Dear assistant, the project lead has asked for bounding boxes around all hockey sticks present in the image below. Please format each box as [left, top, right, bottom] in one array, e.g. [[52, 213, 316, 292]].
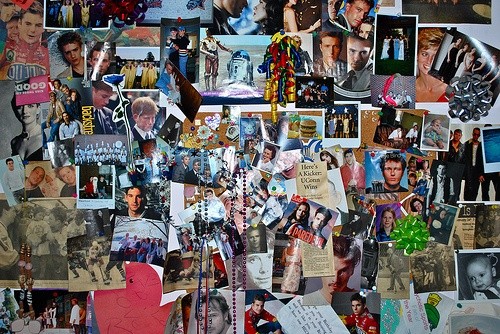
[[386, 264, 398, 295], [83, 259, 95, 282], [99, 266, 113, 283]]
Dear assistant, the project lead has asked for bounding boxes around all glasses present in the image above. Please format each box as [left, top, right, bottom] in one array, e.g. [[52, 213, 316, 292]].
[[472, 133, 481, 137]]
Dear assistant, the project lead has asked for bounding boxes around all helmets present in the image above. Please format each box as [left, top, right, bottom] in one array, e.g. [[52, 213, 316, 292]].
[[92, 241, 97, 248]]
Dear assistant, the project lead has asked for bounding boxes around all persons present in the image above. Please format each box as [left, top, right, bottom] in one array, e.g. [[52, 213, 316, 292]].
[[0, 0, 500, 334]]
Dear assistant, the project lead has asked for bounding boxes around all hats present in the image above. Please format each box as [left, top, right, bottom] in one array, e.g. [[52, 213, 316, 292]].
[[178, 26, 186, 30]]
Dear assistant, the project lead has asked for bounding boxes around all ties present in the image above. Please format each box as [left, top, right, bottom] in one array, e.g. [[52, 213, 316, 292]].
[[145, 134, 149, 140], [99, 111, 103, 127], [341, 70, 356, 91]]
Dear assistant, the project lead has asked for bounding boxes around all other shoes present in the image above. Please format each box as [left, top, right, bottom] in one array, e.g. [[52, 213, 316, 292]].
[[92, 278, 97, 282], [73, 274, 80, 279], [121, 279, 126, 283]]
